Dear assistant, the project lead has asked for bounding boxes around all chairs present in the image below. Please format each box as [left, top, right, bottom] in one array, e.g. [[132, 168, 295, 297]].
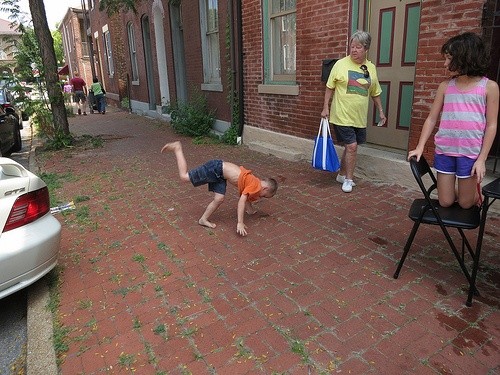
[[393, 154, 480, 296]]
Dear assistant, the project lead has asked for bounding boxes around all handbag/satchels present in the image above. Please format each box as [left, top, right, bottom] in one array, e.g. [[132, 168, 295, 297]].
[[311, 116, 339, 173], [100, 83, 106, 94]]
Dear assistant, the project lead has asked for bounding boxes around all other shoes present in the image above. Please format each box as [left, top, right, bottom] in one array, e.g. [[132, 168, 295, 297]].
[[83, 111, 88, 115], [78, 109, 81, 115], [473, 174, 482, 209]]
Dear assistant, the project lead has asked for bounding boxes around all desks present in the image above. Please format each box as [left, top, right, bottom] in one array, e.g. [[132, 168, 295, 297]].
[[466, 178, 500, 307]]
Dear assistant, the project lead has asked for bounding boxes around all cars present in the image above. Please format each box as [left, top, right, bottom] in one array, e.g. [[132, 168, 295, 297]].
[[0, 157, 62, 300], [0, 76, 33, 157]]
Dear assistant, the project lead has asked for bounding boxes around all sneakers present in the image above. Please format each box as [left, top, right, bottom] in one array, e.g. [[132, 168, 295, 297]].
[[336, 175, 354, 193]]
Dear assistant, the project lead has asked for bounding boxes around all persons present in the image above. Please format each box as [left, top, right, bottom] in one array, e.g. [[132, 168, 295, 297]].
[[89, 78, 106, 115], [407, 32, 500, 209], [321, 30, 386, 193], [64, 81, 72, 103], [162, 140, 278, 237], [70, 72, 87, 116]]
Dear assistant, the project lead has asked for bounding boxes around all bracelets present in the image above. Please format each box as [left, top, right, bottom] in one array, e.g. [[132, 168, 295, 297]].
[[378, 109, 383, 112]]
